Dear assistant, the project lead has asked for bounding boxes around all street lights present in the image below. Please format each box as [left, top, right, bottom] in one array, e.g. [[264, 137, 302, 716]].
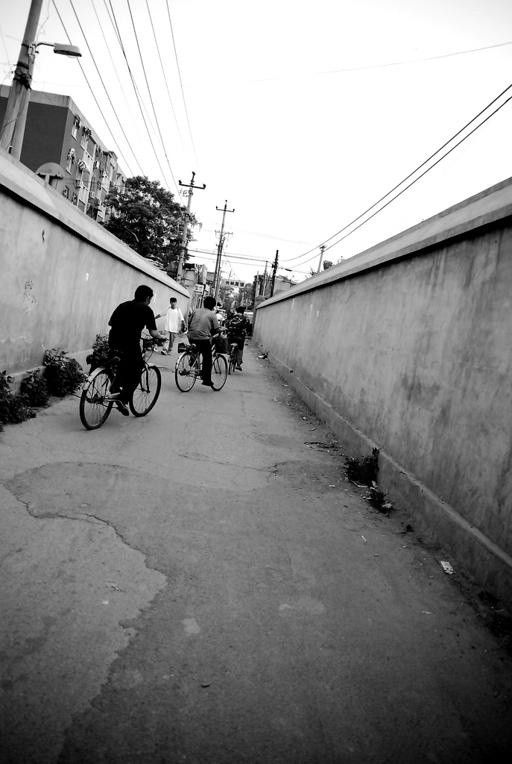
[[2, 42, 82, 156]]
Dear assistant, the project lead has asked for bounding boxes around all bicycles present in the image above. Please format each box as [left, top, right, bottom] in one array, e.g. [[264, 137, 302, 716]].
[[174, 328, 228, 393], [79, 337, 167, 431], [228, 342, 238, 375]]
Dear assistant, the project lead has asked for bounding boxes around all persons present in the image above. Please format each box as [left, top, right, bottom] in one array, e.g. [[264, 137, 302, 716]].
[[241, 306, 256, 345], [106, 284, 167, 417], [215, 310, 225, 327], [222, 305, 253, 371], [185, 296, 229, 386], [154, 297, 186, 355]]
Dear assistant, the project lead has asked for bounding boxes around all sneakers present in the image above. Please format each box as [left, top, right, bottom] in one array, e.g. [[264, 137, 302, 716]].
[[116, 399, 129, 416], [203, 379, 213, 386], [161, 349, 171, 355], [236, 366, 242, 371]]
